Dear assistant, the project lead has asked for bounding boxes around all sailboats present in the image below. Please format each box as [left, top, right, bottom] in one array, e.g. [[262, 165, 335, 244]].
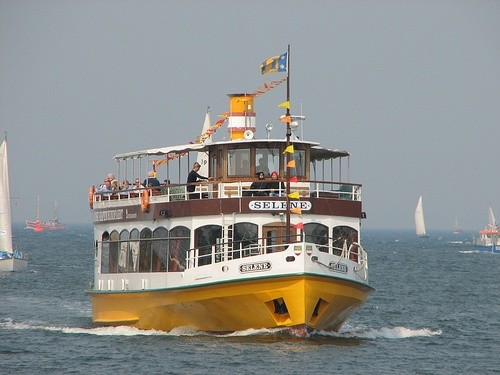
[[413, 193, 431, 239], [0, 129, 30, 273]]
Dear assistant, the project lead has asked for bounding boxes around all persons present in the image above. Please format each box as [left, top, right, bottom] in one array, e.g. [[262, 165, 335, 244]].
[[255, 158, 270, 176], [266, 171, 285, 197], [95, 171, 170, 197], [187, 162, 213, 199], [250, 171, 270, 196]]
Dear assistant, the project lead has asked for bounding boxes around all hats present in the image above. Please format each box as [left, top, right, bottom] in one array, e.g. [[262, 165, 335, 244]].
[[272, 171, 278, 176]]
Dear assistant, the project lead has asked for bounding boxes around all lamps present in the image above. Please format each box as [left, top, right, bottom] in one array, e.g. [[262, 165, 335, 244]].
[[267, 122, 273, 140]]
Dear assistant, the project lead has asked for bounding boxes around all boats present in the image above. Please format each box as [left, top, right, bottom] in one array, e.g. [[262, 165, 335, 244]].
[[25, 195, 64, 233], [81, 42, 377, 335], [471, 222, 500, 253]]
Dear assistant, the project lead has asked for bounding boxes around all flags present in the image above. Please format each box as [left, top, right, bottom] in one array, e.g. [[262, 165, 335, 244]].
[[260, 52, 288, 75]]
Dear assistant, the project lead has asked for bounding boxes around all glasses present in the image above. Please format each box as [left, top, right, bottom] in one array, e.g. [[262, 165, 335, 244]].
[[136, 182, 139, 183]]
